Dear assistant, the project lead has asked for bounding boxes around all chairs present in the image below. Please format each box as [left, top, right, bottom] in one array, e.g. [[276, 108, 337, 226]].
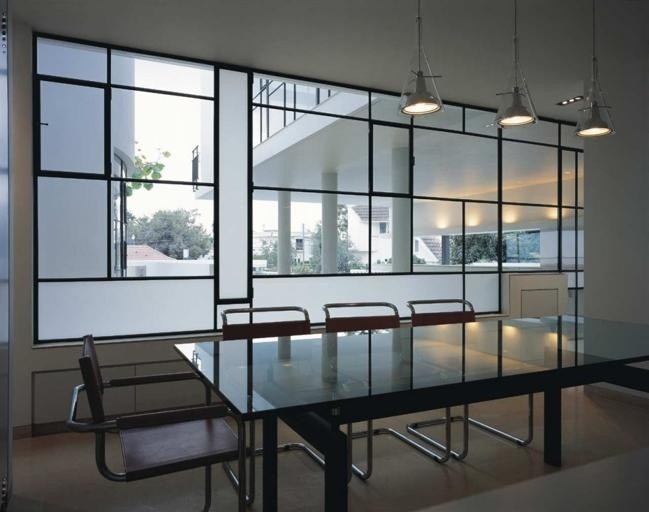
[[69, 331, 244, 512], [221, 306, 352, 505], [405, 299, 534, 461], [322, 302, 451, 481]]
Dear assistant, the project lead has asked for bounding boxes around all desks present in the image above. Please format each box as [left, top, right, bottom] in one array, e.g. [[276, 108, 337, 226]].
[[172, 315, 647, 512]]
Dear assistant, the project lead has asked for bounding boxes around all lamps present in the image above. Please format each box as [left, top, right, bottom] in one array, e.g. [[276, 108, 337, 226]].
[[572, 1, 614, 138], [396, 0, 443, 117], [499, 1, 539, 128]]
